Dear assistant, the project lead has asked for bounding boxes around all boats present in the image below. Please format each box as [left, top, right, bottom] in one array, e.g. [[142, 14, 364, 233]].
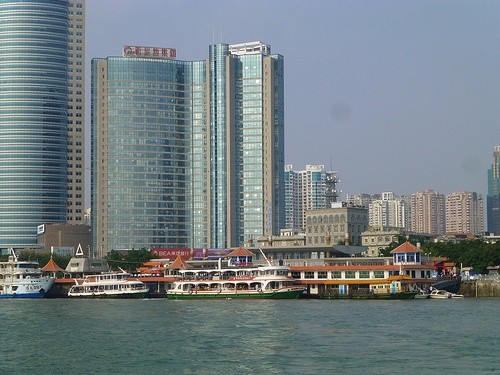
[[0, 249, 57, 298], [428, 290, 465, 300], [64, 273, 152, 299], [281, 263, 422, 301], [165, 268, 308, 299]]
